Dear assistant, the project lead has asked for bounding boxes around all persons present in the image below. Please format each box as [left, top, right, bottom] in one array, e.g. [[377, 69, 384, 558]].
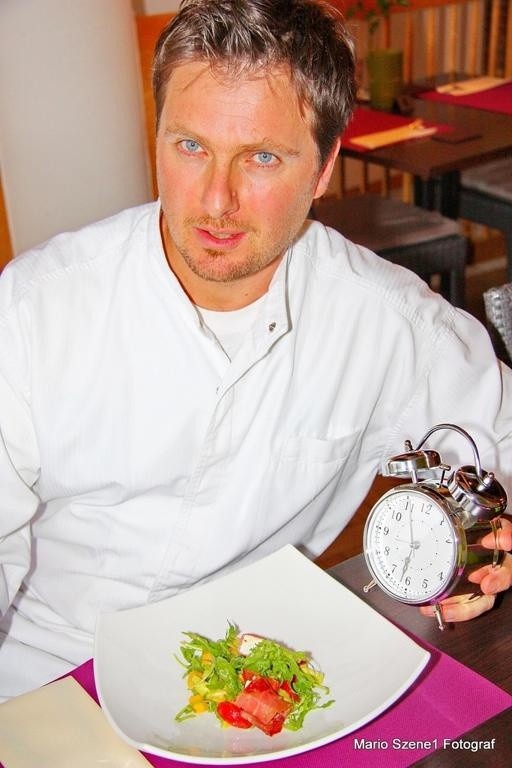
[[0, 0, 512, 705]]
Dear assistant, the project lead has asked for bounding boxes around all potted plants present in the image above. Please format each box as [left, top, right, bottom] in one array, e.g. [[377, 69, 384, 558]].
[[348, 0, 410, 112]]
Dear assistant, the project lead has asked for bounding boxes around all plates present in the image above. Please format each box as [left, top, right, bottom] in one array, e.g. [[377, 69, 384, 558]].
[[94, 542, 433, 765]]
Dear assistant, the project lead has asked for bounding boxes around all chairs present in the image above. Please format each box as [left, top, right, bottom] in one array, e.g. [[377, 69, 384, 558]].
[[312, 193, 474, 312], [424, 158, 512, 288]]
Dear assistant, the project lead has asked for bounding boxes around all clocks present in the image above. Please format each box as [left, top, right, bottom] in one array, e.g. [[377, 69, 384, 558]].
[[363, 423, 507, 631]]
